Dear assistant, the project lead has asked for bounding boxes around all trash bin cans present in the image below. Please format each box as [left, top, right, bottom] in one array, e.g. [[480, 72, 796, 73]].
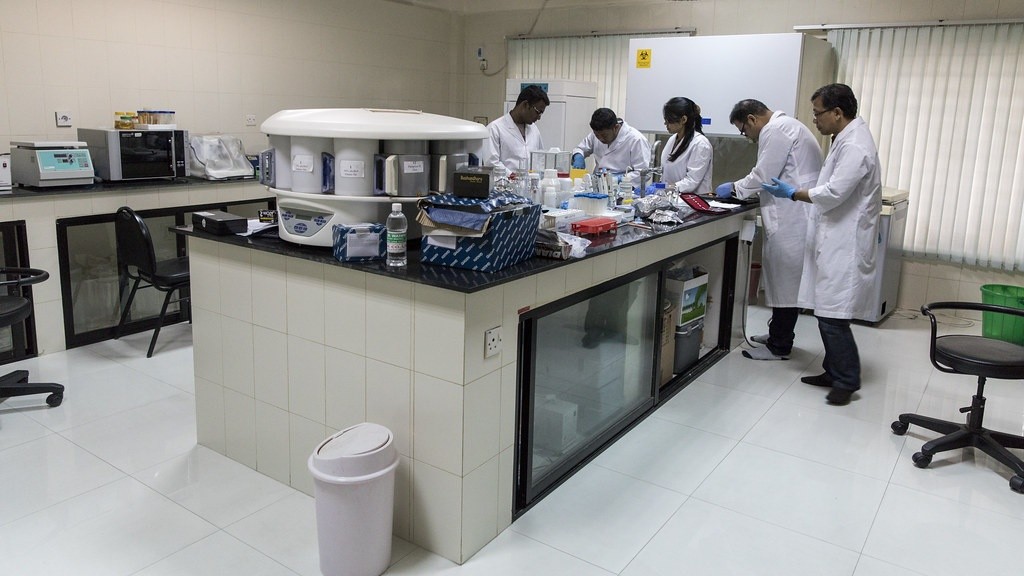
[[979, 283, 1024, 347], [307, 421, 400, 576]]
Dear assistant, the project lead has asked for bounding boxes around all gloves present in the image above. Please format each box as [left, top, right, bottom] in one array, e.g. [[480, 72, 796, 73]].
[[634, 186, 641, 195], [714, 182, 734, 198], [762, 177, 796, 201], [572, 154, 586, 169], [617, 175, 622, 185], [646, 181, 656, 195]]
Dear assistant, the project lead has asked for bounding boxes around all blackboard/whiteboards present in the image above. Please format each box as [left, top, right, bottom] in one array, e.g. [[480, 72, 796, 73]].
[[625, 33, 805, 139]]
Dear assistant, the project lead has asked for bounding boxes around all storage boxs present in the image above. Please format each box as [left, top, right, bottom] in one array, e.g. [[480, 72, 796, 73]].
[[665, 265, 709, 327], [192, 210, 247, 234], [415, 189, 542, 273], [658, 308, 676, 388], [332, 223, 387, 262]]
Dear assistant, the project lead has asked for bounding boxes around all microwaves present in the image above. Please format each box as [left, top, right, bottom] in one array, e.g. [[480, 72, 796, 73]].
[[77, 126, 187, 182]]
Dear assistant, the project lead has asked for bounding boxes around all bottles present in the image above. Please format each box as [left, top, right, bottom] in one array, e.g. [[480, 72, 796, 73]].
[[479, 167, 583, 209], [114, 112, 139, 130], [385, 203, 408, 267], [620, 178, 632, 201], [654, 183, 666, 200]]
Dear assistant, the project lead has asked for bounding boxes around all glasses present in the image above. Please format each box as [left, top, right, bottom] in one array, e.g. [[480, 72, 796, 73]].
[[740, 113, 755, 137], [663, 116, 682, 124], [812, 106, 844, 120], [532, 104, 543, 117]]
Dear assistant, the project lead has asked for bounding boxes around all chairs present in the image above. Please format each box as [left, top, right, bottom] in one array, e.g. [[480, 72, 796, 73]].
[[889, 302, 1024, 493], [0, 265, 65, 407], [114, 206, 191, 357]]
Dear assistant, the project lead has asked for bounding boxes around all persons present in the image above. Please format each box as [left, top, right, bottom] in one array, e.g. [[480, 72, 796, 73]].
[[715, 98, 826, 361], [760, 84, 882, 406], [483, 85, 550, 180], [581, 274, 640, 350], [659, 97, 714, 194], [571, 108, 652, 188]]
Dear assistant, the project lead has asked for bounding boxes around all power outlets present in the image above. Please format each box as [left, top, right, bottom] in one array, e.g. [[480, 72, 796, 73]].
[[55, 111, 72, 126], [474, 117, 488, 126], [484, 326, 503, 359], [245, 115, 256, 125], [480, 61, 487, 69]]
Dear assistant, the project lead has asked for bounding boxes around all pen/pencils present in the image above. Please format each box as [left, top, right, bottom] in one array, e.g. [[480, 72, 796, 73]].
[[627, 223, 652, 230]]
